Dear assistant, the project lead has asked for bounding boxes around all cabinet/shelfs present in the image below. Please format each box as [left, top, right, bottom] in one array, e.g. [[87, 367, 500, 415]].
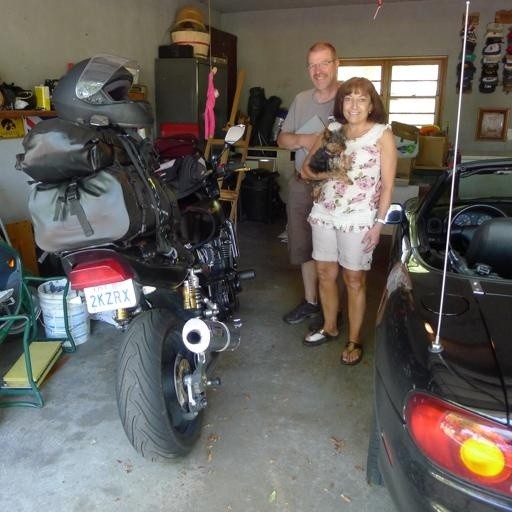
[[155, 59, 228, 143]]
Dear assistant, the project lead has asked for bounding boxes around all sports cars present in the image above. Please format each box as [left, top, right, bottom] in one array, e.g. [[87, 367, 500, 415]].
[[366, 159, 511, 512]]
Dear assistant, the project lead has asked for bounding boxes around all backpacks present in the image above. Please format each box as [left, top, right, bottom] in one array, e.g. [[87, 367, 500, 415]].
[[16, 119, 150, 178]]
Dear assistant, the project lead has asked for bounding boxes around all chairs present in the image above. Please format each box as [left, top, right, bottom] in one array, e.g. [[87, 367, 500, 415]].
[[0, 238, 77, 410], [468, 216, 511, 279]]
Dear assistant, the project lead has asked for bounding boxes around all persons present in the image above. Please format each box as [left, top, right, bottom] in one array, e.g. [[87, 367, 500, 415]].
[[276, 43, 346, 331], [302, 76, 396, 366]]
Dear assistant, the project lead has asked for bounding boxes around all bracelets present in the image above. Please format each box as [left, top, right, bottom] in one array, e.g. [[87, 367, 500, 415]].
[[375, 217, 386, 225]]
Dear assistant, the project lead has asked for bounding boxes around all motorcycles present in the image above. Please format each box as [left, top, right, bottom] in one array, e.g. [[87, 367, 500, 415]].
[[56, 123, 257, 464]]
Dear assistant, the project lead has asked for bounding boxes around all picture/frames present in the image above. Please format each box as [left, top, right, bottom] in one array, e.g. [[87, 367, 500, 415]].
[[477, 107, 510, 141]]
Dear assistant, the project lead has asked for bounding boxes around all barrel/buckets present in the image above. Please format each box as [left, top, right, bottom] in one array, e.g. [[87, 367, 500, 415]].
[[38, 280, 91, 349]]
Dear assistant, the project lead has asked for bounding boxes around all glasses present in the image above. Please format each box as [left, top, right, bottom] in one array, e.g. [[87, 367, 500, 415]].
[[307, 59, 335, 71]]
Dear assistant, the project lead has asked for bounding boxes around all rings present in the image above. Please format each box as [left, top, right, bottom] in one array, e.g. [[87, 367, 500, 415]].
[[373, 245, 376, 248]]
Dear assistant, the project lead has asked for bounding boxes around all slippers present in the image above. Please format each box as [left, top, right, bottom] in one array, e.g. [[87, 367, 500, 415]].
[[340, 341, 364, 365], [303, 328, 340, 347]]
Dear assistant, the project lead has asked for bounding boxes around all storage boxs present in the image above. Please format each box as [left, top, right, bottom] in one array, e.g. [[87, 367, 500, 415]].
[[391, 122, 450, 179]]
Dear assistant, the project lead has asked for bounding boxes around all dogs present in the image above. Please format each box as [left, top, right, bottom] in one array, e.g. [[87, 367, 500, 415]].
[[301, 127, 354, 202]]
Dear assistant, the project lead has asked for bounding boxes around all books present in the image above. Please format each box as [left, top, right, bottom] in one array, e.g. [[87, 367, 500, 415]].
[[294, 114, 325, 156]]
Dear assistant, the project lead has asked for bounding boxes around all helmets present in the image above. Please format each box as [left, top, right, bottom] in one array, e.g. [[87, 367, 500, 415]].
[[50, 52, 154, 132]]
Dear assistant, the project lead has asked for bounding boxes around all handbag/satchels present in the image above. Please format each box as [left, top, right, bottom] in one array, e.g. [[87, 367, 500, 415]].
[[28, 165, 182, 257]]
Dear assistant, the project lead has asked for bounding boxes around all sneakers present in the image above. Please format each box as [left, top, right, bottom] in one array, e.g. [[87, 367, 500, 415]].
[[309, 307, 345, 331], [283, 295, 322, 325]]
